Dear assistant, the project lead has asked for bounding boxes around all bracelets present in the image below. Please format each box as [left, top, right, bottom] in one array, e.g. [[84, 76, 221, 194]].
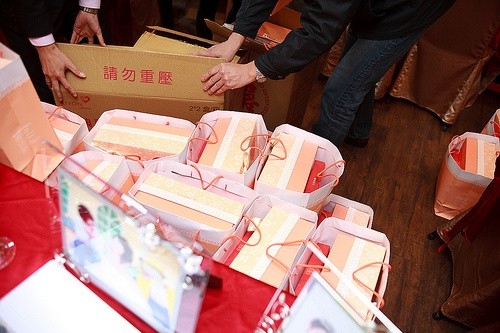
[[80, 6, 98, 14]]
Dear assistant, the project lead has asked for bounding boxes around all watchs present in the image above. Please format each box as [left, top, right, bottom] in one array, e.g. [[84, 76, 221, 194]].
[[256, 68, 266, 83]]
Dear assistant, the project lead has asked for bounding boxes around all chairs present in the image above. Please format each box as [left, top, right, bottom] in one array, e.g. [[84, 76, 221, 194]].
[[320, 22, 397, 104], [384, 0, 500, 134], [426, 174, 500, 333]]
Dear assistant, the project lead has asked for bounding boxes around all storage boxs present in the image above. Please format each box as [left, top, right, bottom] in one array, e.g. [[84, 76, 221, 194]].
[[46, 112, 81, 143], [228, 207, 315, 291], [77, 160, 118, 193], [50, 41, 229, 128], [204, 17, 315, 131], [304, 159, 326, 194], [133, 26, 250, 112], [133, 171, 245, 231], [331, 203, 370, 229], [459, 137, 496, 179], [256, 133, 319, 192], [321, 232, 388, 322], [265, 0, 305, 31], [224, 230, 256, 266], [93, 117, 193, 153], [196, 117, 259, 174], [317, 209, 333, 228], [494, 110, 500, 133], [295, 242, 331, 296]]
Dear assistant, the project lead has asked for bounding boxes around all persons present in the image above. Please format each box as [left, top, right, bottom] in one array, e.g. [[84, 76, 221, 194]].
[[187, 0, 456, 151], [73, 205, 100, 269], [111, 234, 132, 263], [0, 0, 106, 101]]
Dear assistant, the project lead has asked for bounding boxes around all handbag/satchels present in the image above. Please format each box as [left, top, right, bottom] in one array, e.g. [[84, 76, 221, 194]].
[[0, 44, 395, 333], [433, 108, 500, 220]]
